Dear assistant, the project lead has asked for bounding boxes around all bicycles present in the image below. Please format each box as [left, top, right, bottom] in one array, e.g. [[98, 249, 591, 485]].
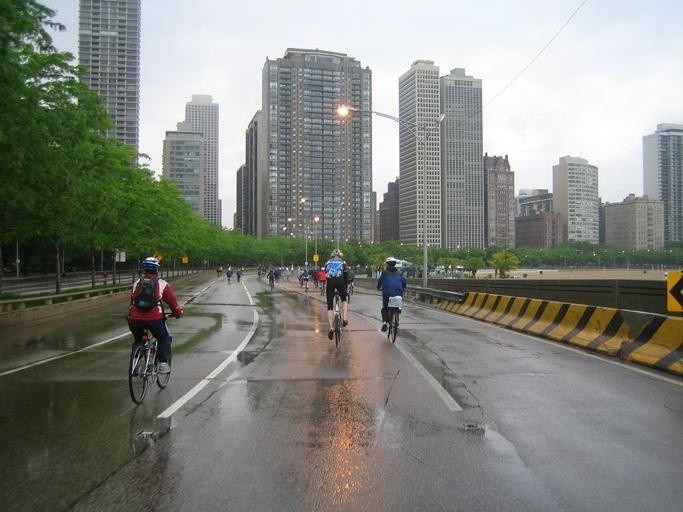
[[268, 279, 276, 292], [129, 313, 174, 404], [379, 289, 402, 342], [228, 277, 232, 284], [328, 288, 347, 349], [300, 278, 354, 303]]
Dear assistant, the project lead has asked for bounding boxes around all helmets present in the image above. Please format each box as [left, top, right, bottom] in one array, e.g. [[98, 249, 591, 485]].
[[330, 249, 344, 260], [384, 256, 398, 264], [141, 256, 161, 273], [320, 267, 325, 271]]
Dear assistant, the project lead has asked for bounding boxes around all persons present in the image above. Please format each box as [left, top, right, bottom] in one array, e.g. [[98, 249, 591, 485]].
[[213, 262, 478, 295], [124, 257, 184, 375], [322, 248, 350, 340], [376, 257, 407, 332]]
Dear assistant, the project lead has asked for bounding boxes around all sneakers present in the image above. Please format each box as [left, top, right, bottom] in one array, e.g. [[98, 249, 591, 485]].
[[328, 320, 348, 340], [159, 362, 171, 374], [381, 324, 388, 331]]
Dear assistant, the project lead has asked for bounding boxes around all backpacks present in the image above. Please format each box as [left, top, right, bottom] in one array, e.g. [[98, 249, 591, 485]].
[[132, 277, 162, 311], [324, 261, 343, 279]]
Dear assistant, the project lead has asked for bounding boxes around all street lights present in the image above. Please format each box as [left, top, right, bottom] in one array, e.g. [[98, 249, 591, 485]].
[[338, 106, 428, 288], [288, 218, 308, 269], [302, 198, 340, 250]]
